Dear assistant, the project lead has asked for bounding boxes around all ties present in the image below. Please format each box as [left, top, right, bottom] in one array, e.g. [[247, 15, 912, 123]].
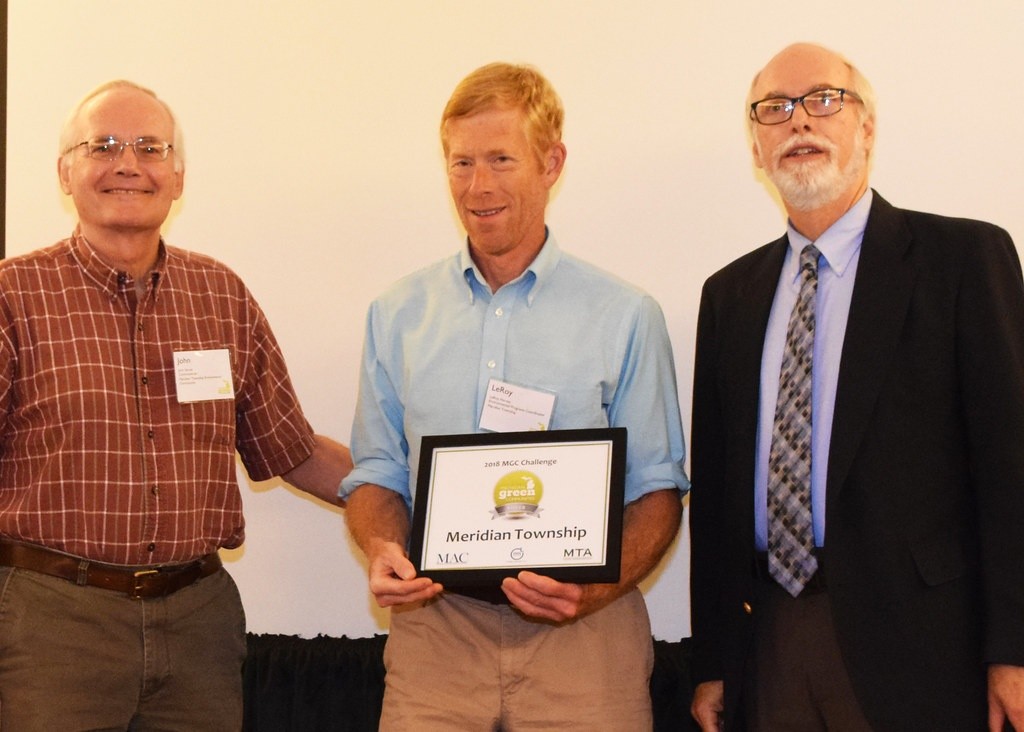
[[766, 245, 823, 597]]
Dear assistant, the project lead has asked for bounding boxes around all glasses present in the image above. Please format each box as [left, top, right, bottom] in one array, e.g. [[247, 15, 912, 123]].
[[749, 85, 857, 125], [61, 135, 180, 164]]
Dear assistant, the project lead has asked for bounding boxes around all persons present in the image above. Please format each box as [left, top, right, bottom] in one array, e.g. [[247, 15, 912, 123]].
[[0, 81, 353, 732], [690, 42, 1024, 732], [337, 63, 691, 732]]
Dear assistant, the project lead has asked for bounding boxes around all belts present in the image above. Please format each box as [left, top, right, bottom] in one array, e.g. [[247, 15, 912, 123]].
[[0, 540, 220, 600]]
